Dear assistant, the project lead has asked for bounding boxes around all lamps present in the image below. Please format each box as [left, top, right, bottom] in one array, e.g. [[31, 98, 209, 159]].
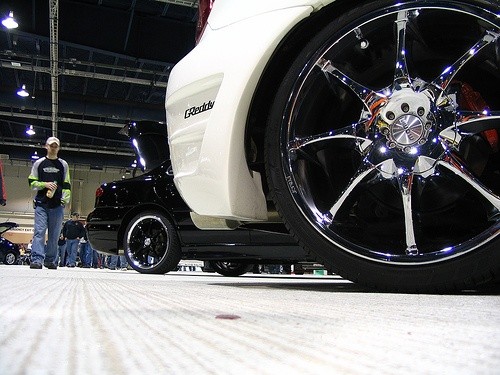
[[31, 151, 40, 159], [17, 83, 29, 96], [26, 125, 35, 135], [1, 10, 18, 29]]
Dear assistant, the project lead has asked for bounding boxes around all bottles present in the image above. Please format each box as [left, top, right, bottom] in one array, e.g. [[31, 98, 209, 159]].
[[46, 181, 58, 198]]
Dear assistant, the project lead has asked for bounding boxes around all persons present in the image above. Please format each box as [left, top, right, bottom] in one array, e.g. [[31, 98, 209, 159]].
[[63, 212, 87, 268], [16, 220, 336, 275], [27, 136, 72, 269]]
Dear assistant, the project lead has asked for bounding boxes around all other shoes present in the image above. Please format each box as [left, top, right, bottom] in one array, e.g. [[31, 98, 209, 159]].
[[44, 260, 57, 269], [30, 261, 42, 269]]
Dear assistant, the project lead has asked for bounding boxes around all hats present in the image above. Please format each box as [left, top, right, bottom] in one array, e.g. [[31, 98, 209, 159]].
[[46, 136, 60, 147]]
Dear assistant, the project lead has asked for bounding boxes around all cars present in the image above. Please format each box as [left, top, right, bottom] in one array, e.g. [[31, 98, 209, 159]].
[[0, 221, 21, 266], [80, 121, 320, 275], [164, 0, 500, 295]]
